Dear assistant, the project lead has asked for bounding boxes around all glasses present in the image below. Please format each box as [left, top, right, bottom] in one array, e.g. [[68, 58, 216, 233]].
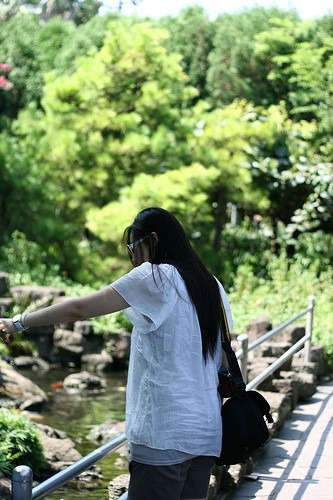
[[127, 236, 149, 257]]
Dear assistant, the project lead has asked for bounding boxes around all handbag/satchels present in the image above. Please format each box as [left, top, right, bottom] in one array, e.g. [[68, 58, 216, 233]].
[[216, 391, 273, 467]]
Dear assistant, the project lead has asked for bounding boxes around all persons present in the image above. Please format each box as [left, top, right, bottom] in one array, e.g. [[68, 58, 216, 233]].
[[0, 208, 233, 500]]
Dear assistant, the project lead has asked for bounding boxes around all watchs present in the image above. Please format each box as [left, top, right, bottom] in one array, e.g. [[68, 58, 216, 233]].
[[12, 314, 25, 331]]
